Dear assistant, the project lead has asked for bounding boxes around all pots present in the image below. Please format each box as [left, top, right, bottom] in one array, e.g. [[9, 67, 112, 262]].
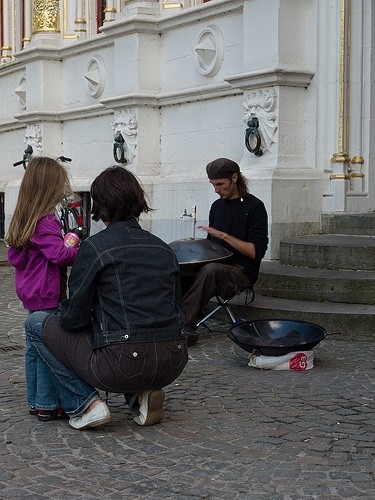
[[228, 319, 342, 357]]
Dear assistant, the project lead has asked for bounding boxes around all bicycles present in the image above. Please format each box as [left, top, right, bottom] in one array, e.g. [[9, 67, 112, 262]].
[[13, 155, 90, 242]]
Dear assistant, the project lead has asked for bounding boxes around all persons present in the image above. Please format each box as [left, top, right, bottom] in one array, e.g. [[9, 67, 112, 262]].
[[179, 158, 269, 347], [5, 156, 81, 421], [24, 165, 188, 430]]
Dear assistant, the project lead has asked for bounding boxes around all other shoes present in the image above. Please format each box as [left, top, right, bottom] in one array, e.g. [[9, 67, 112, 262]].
[[180, 326, 199, 347], [38, 408, 69, 421], [132, 389, 165, 426], [30, 407, 38, 414], [69, 401, 112, 430]]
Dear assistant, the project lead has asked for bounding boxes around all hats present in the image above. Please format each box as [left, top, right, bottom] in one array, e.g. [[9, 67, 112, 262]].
[[206, 158, 240, 178]]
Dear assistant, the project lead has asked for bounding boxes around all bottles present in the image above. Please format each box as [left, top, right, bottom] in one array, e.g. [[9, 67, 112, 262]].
[[64, 227, 84, 246]]
